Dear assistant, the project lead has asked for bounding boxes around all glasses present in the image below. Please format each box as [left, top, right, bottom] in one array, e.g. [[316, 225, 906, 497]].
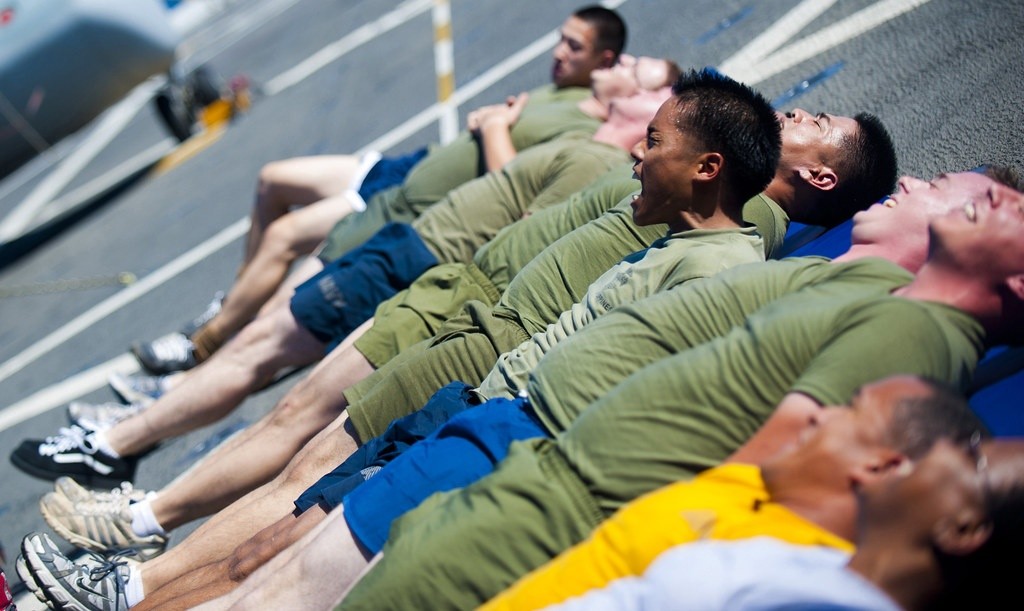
[[970, 430, 1001, 515]]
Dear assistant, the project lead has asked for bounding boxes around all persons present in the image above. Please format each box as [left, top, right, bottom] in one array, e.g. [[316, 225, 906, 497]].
[[476, 373, 973, 609], [36, 106, 899, 565], [16, 67, 787, 611], [333, 183, 1024, 610], [64, 51, 684, 436], [133, 4, 630, 375], [10, 84, 677, 488], [184, 162, 1018, 611], [548, 435, 1024, 607]]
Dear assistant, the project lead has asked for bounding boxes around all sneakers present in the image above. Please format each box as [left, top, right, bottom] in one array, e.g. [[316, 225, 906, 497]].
[[68, 401, 137, 430], [16, 531, 128, 611], [56, 476, 152, 503], [129, 331, 199, 372], [40, 492, 170, 563], [182, 289, 224, 338], [11, 430, 134, 487], [109, 368, 183, 407]]
